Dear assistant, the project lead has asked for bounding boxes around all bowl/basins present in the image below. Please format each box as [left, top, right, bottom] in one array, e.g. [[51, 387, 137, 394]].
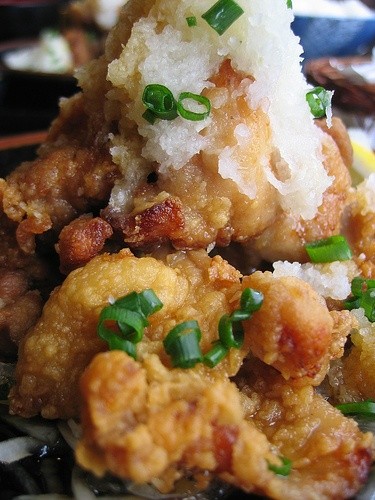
[[0, 39, 81, 104], [0, 130, 375, 500]]
